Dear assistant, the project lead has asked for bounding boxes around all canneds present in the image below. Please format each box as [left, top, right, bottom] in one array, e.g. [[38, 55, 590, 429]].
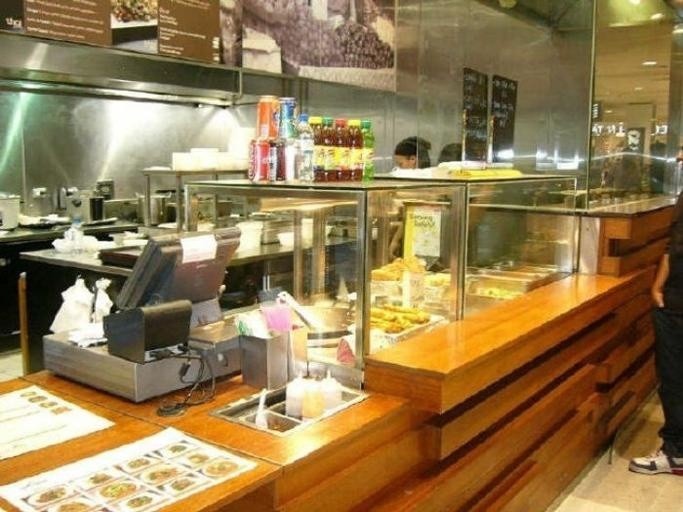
[[249, 95, 296, 182]]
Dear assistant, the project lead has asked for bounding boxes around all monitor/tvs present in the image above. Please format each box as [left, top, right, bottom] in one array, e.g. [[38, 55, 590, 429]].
[[116, 228, 241, 311]]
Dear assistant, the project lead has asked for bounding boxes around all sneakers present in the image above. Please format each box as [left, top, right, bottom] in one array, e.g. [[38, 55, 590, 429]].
[[628, 449, 681, 477]]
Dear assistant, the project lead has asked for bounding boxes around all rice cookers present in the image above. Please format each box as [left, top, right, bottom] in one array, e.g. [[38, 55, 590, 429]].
[[0, 191, 25, 231]]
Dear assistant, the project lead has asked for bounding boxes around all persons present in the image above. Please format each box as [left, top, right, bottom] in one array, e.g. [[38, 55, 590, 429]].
[[629, 145, 683, 475], [388, 136, 430, 262]]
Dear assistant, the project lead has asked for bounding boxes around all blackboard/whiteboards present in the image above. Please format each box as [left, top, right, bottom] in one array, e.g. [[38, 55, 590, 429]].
[[462, 67, 488, 163], [492, 75, 518, 163]]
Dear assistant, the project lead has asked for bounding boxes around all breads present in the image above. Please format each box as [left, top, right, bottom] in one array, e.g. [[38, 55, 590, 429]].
[[362, 258, 472, 334]]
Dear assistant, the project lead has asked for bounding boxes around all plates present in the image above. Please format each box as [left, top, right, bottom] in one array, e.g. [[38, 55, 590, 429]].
[[20, 390, 68, 413], [109, 3, 163, 30], [30, 441, 238, 512]]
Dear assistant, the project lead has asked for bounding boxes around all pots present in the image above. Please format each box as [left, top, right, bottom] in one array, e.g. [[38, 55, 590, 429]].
[[134, 194, 172, 226]]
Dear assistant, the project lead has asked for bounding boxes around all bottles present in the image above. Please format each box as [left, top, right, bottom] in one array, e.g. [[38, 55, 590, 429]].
[[72, 214, 80, 228], [91, 197, 103, 221], [52, 187, 57, 210], [59, 186, 66, 210], [248, 94, 378, 181]]
